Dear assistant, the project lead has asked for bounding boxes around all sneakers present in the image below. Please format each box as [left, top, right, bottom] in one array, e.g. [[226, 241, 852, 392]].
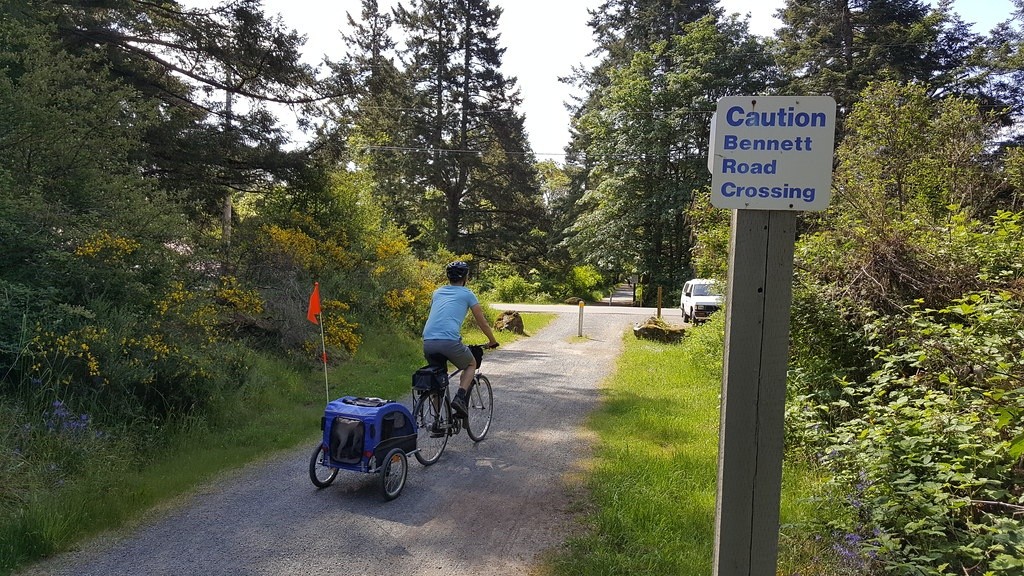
[[432, 417, 446, 434], [451, 396, 469, 417]]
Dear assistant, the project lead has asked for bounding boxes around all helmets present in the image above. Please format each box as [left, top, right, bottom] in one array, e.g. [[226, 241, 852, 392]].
[[447, 261, 468, 278]]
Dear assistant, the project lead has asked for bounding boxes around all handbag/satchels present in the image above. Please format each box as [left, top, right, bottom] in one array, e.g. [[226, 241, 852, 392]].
[[411, 364, 449, 389]]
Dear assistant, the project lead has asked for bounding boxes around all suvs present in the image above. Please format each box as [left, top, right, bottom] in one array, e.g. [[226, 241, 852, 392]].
[[679, 278, 726, 327]]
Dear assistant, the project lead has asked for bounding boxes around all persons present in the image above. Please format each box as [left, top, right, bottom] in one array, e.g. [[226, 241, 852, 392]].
[[422, 262, 497, 437]]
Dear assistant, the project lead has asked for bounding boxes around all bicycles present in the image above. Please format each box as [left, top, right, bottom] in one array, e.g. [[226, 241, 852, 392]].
[[411, 343, 500, 466]]
